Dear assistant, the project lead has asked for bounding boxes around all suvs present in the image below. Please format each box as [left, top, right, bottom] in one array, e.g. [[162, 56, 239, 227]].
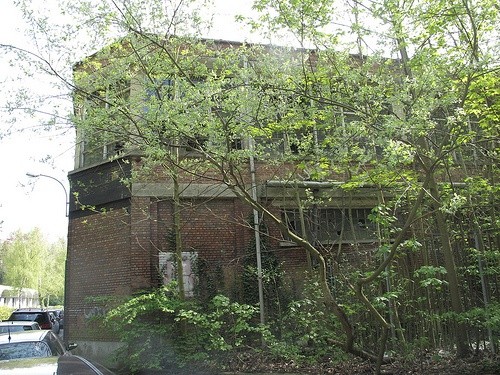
[[8, 309, 61, 332]]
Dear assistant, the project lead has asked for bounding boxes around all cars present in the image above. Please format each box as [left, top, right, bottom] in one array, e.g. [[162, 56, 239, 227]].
[[1, 321, 42, 335], [45, 309, 61, 327], [0, 356, 118, 375], [55, 310, 64, 328], [0, 329, 77, 362]]
